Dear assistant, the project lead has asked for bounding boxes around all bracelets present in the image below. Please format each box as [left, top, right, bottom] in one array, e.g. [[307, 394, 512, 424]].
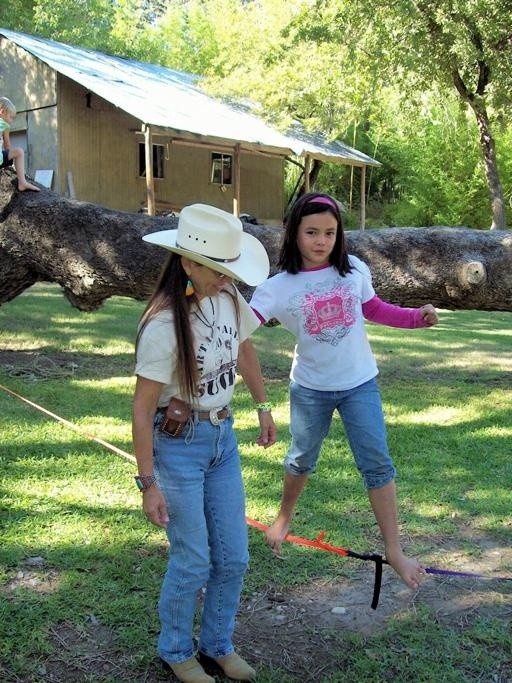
[[135, 474, 156, 492], [255, 401, 274, 412]]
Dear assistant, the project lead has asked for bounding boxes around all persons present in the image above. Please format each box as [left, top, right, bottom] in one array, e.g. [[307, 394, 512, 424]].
[[247, 193, 437, 587], [0, 96, 40, 191], [132, 201, 276, 683]]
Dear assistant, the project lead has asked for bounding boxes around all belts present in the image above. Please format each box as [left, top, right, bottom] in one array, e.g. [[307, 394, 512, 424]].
[[156, 407, 230, 421]]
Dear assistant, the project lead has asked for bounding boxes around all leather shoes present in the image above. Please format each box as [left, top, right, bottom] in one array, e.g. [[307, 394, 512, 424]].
[[214, 651, 256, 680], [169, 655, 216, 683]]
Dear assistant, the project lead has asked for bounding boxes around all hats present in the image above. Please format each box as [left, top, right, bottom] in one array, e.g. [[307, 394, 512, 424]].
[[140, 202, 270, 287]]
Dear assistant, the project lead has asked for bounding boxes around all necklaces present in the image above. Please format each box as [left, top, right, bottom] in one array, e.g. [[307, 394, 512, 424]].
[[194, 295, 215, 340]]
